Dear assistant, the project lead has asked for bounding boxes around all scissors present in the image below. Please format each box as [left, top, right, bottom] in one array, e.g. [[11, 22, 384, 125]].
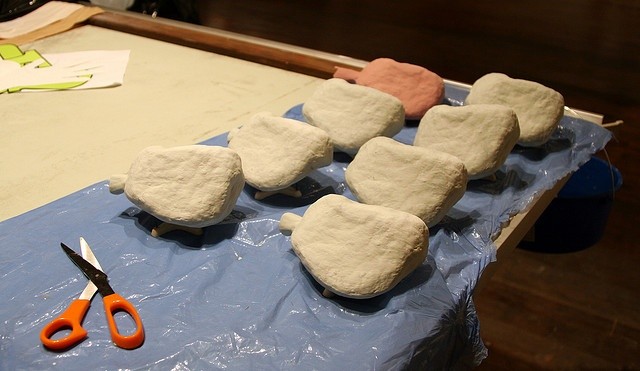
[[39, 238, 144, 351]]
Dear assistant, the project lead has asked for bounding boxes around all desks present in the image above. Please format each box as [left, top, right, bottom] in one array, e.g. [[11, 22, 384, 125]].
[[0, 1, 604, 371]]
